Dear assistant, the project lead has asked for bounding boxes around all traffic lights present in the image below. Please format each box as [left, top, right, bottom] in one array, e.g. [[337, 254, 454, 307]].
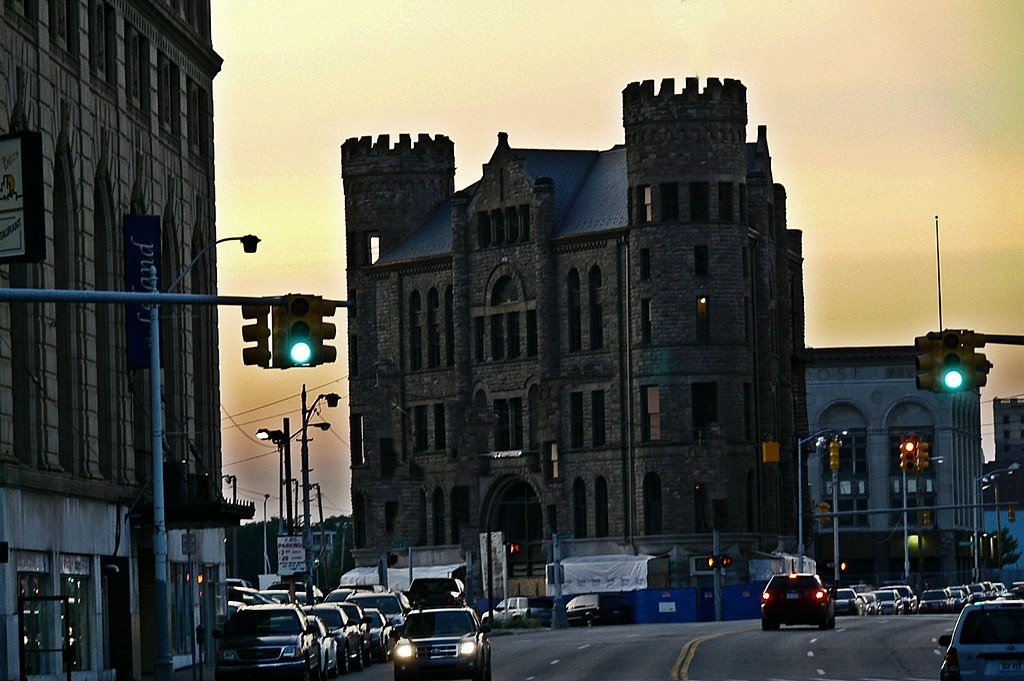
[[900, 439, 929, 473], [914, 330, 989, 392], [242, 290, 336, 370], [819, 503, 830, 522], [708, 555, 732, 568], [504, 540, 521, 555]]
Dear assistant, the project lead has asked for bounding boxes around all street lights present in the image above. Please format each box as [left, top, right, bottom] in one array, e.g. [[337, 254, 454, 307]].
[[148, 235, 262, 680], [301, 382, 343, 605], [902, 454, 945, 584], [278, 422, 332, 535], [979, 462, 1021, 570], [262, 493, 271, 574], [797, 425, 848, 573]]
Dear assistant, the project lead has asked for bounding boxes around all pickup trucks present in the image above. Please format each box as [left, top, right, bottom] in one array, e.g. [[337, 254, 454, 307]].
[[480, 596, 551, 626]]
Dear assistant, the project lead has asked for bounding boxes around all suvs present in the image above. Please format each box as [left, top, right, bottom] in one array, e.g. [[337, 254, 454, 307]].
[[761, 574, 835, 631], [849, 581, 1024, 617], [836, 588, 860, 616], [565, 592, 631, 628], [393, 605, 494, 681], [408, 577, 465, 611], [939, 599, 1024, 681], [225, 577, 411, 681]]
